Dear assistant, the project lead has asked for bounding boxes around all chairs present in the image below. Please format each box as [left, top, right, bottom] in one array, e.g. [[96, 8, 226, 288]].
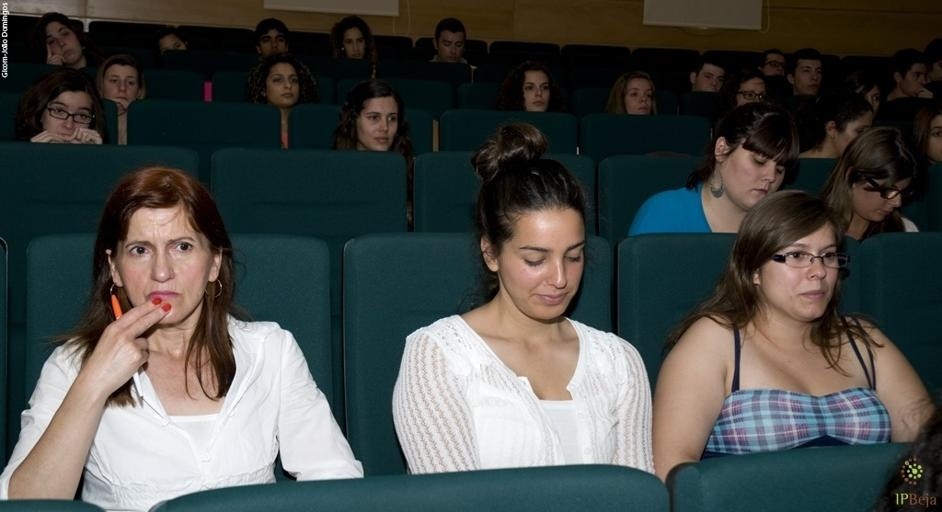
[[2, 16, 942, 235], [619, 234, 858, 464], [28, 245, 338, 476], [856, 232, 941, 408], [344, 241, 612, 475], [146, 464, 669, 512], [667, 444, 906, 512]]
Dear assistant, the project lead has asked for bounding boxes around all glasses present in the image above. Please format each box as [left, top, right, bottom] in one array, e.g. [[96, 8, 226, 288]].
[[48, 106, 95, 126], [737, 89, 767, 101], [763, 60, 785, 68], [869, 178, 916, 199], [773, 250, 849, 269]]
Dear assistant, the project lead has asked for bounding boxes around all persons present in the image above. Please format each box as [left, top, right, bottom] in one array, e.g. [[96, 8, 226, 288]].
[[15, 1, 942, 236], [393, 119, 659, 475], [654, 189, 938, 485], [865, 381, 942, 512], [0, 164, 365, 511]]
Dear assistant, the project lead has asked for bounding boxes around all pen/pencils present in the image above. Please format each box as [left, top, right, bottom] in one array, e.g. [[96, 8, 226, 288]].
[[111, 294, 144, 401]]
